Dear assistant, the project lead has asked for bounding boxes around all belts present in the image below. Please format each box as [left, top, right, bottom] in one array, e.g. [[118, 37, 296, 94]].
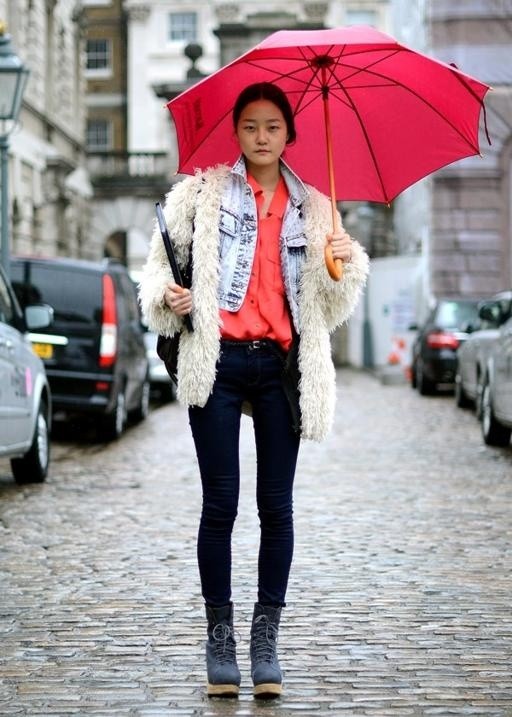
[[220, 338, 277, 351]]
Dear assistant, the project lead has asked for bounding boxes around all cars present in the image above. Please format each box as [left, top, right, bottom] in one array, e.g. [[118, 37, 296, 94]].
[[0, 264, 52, 484], [7, 258, 154, 438], [406, 287, 512, 442]]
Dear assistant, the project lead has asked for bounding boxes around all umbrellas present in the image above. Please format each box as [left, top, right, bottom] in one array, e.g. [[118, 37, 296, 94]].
[[162, 26, 493, 280]]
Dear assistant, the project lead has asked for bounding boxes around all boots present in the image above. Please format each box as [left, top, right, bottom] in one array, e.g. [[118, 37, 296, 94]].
[[250, 602, 282, 697], [205, 600, 241, 697]]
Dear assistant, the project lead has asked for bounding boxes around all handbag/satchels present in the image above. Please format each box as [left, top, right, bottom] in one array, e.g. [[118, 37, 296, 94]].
[[157, 254, 192, 386]]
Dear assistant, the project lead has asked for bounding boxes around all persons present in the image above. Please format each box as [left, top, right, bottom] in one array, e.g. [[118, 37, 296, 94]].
[[137, 82, 370, 697]]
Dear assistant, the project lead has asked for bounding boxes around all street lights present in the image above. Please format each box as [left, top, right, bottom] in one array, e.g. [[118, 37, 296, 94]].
[[0, 28, 29, 284]]
